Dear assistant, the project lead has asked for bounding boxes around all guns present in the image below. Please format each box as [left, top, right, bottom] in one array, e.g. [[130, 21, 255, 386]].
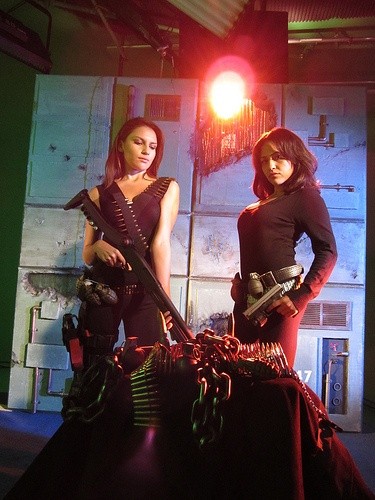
[[242, 281, 284, 328], [64, 189, 195, 341]]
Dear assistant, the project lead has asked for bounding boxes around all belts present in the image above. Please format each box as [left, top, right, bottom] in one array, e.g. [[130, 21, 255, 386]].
[[259, 265, 304, 290]]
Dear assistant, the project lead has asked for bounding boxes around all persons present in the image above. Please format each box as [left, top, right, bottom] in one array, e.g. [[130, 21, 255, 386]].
[[78, 117, 179, 365], [234, 128, 338, 371]]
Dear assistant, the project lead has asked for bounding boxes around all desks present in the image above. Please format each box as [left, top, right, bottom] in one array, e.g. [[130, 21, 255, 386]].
[[0, 348, 375, 500]]
